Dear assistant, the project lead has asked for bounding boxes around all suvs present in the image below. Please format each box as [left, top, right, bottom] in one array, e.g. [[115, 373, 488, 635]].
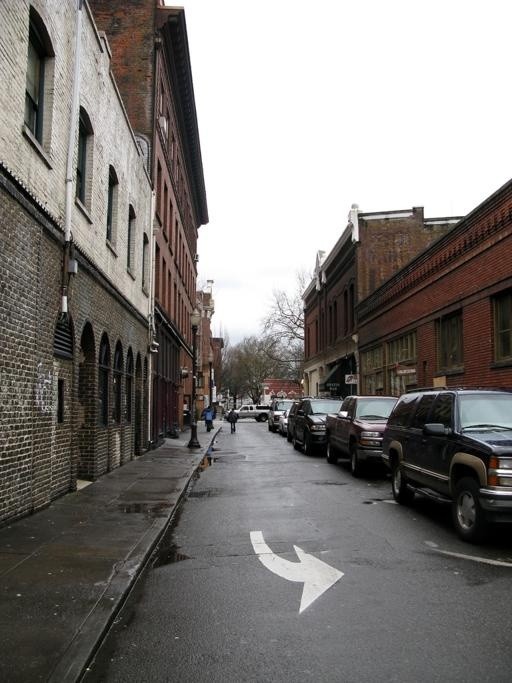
[[292, 398, 345, 456], [287, 401, 299, 443], [268, 398, 292, 433], [224, 404, 271, 422], [380, 384, 512, 544]]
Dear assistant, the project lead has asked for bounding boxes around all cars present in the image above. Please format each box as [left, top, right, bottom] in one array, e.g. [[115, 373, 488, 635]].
[[279, 410, 289, 437]]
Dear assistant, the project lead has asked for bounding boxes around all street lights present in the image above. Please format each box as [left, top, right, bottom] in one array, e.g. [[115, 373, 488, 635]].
[[189, 308, 201, 448], [208, 348, 213, 405]]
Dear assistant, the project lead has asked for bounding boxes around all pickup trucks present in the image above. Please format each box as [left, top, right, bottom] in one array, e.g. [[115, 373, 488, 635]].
[[325, 396, 399, 477]]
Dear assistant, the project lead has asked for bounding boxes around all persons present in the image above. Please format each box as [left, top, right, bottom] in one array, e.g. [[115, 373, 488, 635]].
[[226, 407, 239, 433], [203, 405, 214, 432]]
